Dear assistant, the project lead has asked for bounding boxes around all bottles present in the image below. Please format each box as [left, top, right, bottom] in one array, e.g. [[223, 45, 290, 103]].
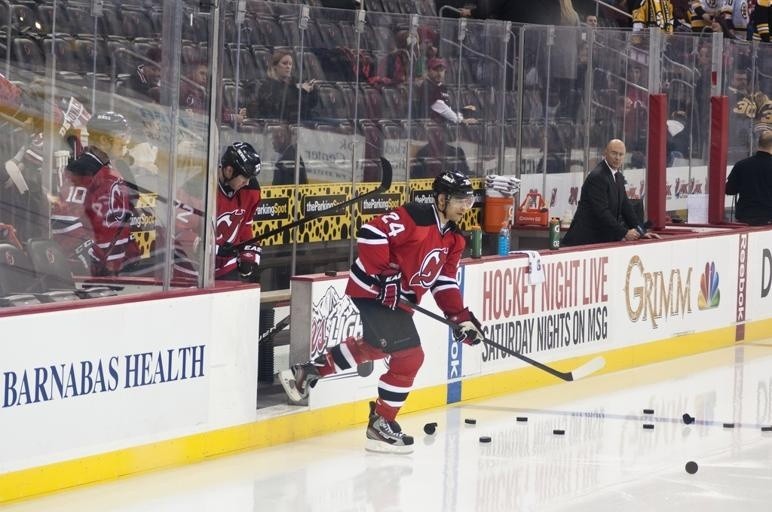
[[498, 222, 512, 257], [470, 221, 483, 257], [549, 216, 561, 250]]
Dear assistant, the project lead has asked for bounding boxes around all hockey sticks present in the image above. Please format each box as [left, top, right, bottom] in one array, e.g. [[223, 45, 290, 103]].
[[398, 296, 605, 381], [219, 157, 393, 255], [5, 160, 30, 250], [97, 161, 138, 276]]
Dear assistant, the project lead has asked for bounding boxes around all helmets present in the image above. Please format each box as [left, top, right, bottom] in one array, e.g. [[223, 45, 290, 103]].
[[432, 170, 474, 200], [225, 141, 261, 176], [87, 111, 131, 134]]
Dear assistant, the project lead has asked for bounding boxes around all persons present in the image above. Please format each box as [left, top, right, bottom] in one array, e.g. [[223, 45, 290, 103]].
[[0, 0, 772, 288], [290, 169, 485, 447]]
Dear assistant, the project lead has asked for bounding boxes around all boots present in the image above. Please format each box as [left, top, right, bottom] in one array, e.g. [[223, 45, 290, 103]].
[[367, 414, 414, 446], [292, 362, 321, 400]]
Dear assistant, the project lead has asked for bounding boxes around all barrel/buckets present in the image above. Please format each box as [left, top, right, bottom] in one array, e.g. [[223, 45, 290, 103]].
[[484, 195, 515, 234]]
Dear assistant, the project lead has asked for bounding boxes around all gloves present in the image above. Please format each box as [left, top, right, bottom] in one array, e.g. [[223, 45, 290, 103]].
[[237, 244, 262, 282], [76, 240, 107, 274], [447, 307, 484, 345], [376, 269, 410, 311], [196, 240, 234, 262]]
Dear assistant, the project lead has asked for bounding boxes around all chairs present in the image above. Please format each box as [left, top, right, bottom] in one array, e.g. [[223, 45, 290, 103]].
[[0, 243, 40, 296], [0, 1, 771, 183], [26, 237, 75, 290]]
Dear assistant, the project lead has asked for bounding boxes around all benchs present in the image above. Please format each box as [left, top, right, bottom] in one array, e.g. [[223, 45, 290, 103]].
[[258, 240, 360, 268], [498, 227, 572, 250]]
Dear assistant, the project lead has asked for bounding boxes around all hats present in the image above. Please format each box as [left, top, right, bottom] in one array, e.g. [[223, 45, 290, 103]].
[[428, 57, 448, 69]]
